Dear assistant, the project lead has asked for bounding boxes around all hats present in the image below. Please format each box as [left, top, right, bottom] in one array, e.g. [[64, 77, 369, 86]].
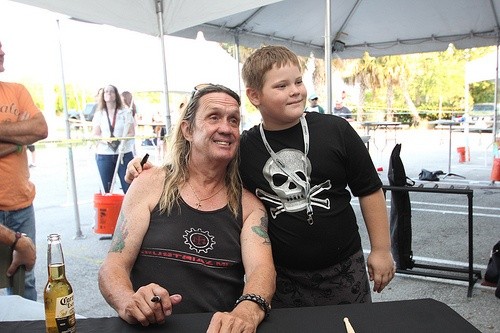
[[309, 93, 319, 101]]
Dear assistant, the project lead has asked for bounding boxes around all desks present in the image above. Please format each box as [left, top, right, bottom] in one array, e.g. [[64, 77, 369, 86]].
[[360, 122, 402, 152], [1, 297, 483, 333]]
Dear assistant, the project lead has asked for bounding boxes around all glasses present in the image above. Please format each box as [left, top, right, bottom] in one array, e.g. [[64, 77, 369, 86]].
[[192, 83, 241, 105]]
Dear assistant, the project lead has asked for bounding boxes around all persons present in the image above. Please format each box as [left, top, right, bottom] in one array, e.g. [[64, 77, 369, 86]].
[[332, 101, 353, 122], [304, 94, 324, 114], [124, 45, 395, 308], [90, 85, 138, 194], [0, 41, 47, 301], [98, 83, 278, 333]]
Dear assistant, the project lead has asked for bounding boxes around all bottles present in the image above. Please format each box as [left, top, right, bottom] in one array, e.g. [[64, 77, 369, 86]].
[[8, 233, 27, 299], [43, 232, 80, 333]]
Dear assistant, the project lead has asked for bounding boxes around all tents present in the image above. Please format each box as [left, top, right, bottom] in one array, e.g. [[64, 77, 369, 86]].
[[463, 50, 500, 163], [9, 0, 500, 240]]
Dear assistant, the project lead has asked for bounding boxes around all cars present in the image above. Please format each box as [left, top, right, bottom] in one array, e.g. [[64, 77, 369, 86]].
[[68, 104, 97, 130], [468, 103, 496, 130]]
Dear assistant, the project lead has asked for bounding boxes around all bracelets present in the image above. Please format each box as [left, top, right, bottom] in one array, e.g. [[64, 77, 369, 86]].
[[234, 294, 272, 319], [17, 144, 23, 153], [12, 232, 21, 247]]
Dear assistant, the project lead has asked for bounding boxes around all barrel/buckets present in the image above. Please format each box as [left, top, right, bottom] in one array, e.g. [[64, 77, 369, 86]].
[[456, 146, 468, 162], [91, 192, 125, 235]]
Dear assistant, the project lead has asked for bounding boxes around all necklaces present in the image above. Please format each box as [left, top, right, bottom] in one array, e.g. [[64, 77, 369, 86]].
[[186, 178, 227, 210], [260, 114, 314, 225]]
